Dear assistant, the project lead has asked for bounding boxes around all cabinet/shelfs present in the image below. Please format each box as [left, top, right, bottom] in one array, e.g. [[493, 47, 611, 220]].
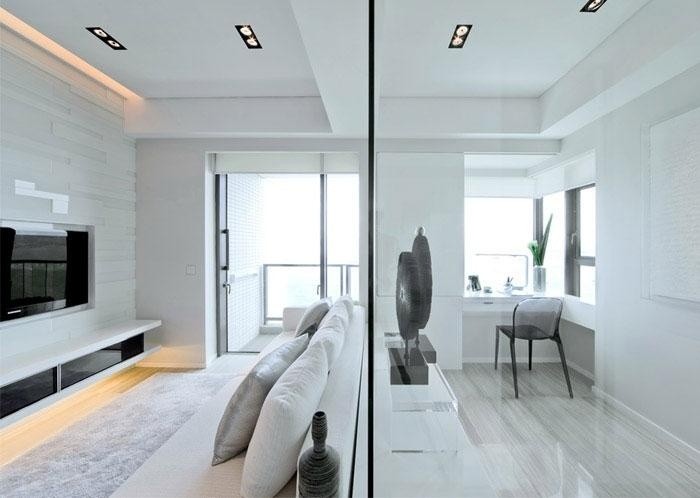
[[0, 320, 163, 430], [384, 333, 497, 498]]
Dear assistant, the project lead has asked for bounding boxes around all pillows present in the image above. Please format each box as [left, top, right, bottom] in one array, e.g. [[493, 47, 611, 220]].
[[241, 340, 328, 498], [307, 315, 344, 373], [316, 301, 348, 335], [337, 296, 352, 318], [294, 298, 329, 338], [211, 333, 309, 467]]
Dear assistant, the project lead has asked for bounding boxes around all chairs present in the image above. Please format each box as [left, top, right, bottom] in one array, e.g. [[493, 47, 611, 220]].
[[494, 296, 573, 399]]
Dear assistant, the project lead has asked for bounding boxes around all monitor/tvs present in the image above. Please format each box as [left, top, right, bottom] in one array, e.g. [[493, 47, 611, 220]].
[[0, 218, 96, 328]]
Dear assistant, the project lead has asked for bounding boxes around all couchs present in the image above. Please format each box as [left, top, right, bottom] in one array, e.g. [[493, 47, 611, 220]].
[[108, 305, 364, 497]]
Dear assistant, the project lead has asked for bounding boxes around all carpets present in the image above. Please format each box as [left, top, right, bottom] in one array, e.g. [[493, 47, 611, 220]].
[[0, 372, 239, 498]]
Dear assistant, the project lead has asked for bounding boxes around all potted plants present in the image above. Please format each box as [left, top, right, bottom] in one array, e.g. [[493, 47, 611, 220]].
[[528, 213, 555, 293]]
